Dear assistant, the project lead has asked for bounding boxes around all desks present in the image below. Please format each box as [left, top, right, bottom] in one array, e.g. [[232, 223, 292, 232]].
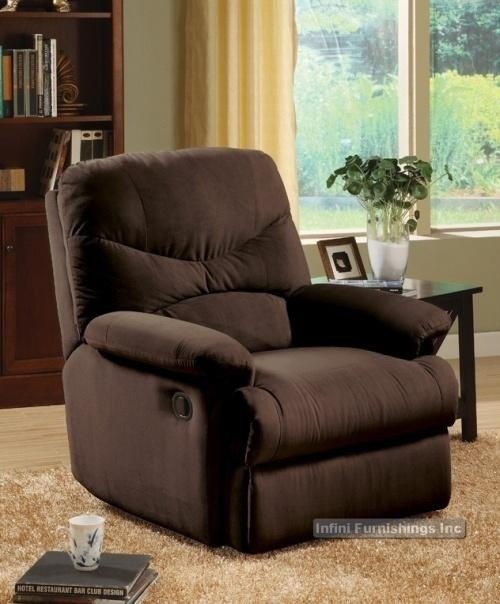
[[312, 276, 483, 443]]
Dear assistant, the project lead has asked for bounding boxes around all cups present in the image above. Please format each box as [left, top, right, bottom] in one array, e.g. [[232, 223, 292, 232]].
[[56, 514, 105, 572]]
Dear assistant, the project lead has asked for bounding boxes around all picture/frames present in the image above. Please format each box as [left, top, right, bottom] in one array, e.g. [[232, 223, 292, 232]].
[[316, 236, 368, 283]]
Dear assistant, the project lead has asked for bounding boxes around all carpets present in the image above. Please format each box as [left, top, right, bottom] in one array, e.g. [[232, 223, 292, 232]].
[[1, 431, 499, 604]]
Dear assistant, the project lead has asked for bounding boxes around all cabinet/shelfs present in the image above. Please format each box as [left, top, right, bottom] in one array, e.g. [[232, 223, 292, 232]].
[[0, 0, 124, 409]]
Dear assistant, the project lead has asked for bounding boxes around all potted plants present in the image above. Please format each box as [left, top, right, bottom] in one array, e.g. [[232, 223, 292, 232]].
[[326, 154, 453, 283]]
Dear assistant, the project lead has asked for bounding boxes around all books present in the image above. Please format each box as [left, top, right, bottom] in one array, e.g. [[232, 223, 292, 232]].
[[0, 30, 111, 201], [401, 288, 417, 297], [12, 550, 155, 599], [11, 568, 159, 604], [330, 277, 404, 293]]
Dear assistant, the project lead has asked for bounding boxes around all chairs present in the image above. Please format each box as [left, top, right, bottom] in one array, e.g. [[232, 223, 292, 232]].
[[44, 146, 460, 554]]
